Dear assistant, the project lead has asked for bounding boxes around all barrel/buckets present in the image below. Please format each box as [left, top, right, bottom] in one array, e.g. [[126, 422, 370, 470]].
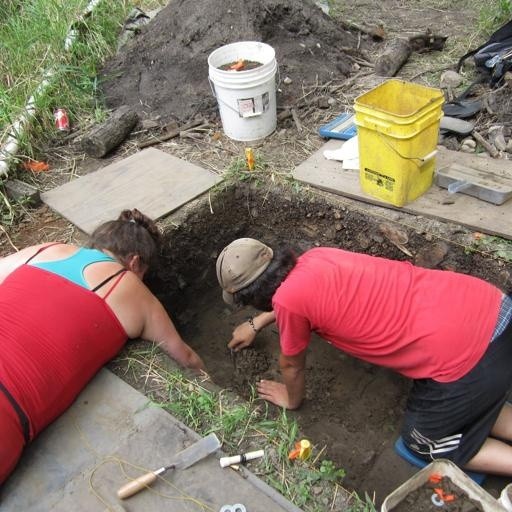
[[207, 41, 283, 141], [353, 77, 445, 207]]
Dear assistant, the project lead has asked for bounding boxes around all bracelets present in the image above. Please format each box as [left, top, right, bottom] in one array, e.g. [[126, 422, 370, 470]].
[[247, 316, 260, 334]]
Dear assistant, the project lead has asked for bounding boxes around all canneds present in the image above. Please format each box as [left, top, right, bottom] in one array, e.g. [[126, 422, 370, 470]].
[[54, 108, 69, 132]]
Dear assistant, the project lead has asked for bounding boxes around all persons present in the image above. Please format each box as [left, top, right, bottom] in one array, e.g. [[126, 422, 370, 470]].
[[214, 237, 512, 477], [0, 209, 209, 512]]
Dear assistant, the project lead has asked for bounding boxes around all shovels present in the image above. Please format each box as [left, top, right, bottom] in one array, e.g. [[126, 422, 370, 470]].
[[117, 432, 220, 499]]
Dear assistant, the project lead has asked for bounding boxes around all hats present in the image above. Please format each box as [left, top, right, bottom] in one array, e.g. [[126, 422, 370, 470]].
[[215, 237, 273, 305]]
